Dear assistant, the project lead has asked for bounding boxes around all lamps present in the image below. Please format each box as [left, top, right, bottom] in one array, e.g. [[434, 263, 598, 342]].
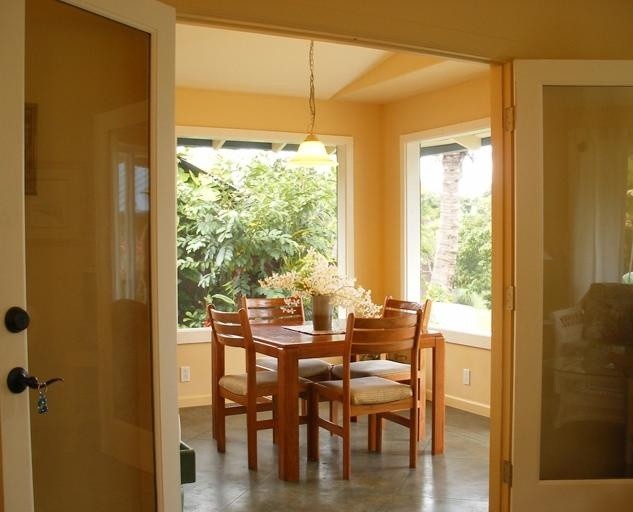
[[292, 39, 340, 167]]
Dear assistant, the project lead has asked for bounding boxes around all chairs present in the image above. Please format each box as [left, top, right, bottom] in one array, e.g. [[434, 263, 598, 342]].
[[307, 309, 423, 480], [548, 282, 632, 425], [207, 304, 318, 470], [239, 293, 332, 417], [329, 297, 432, 443]]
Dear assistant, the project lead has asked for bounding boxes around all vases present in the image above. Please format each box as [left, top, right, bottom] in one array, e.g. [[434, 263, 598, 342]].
[[311, 295, 333, 331]]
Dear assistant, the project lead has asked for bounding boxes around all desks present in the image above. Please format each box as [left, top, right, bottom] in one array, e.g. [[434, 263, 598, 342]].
[[243, 321, 445, 482]]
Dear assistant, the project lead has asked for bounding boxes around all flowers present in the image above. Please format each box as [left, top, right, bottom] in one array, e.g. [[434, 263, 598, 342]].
[[256, 246, 384, 319]]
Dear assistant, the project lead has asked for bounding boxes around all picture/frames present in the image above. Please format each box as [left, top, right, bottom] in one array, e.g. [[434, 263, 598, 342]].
[[25, 103, 38, 196]]
